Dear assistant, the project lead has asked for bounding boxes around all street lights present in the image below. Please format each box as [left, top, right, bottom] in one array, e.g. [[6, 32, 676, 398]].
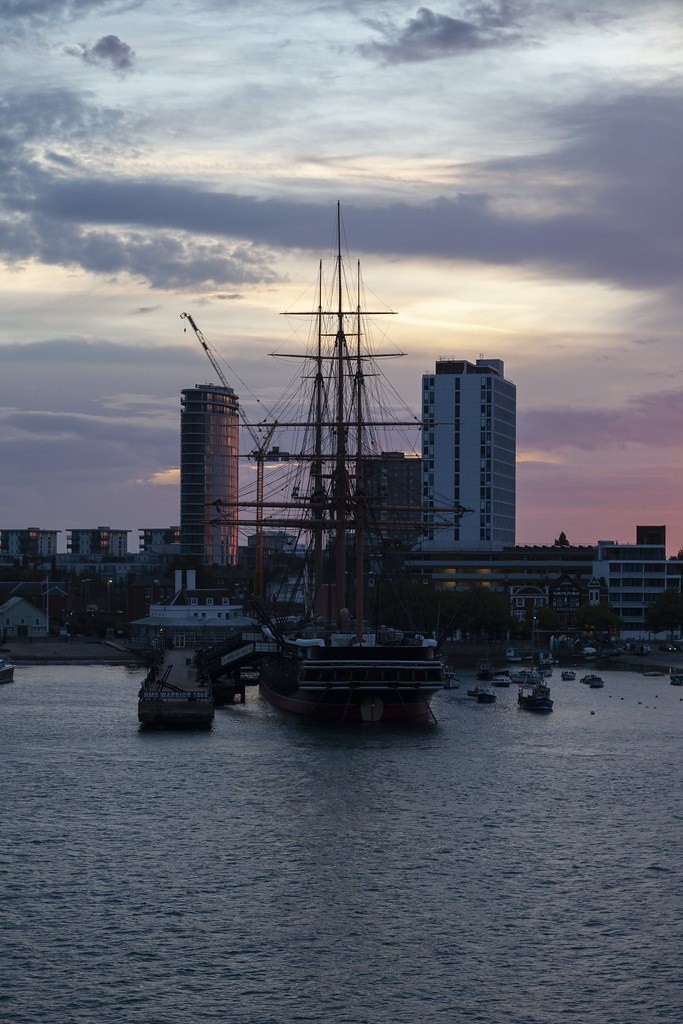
[[532, 615, 537, 671], [108, 579, 113, 613]]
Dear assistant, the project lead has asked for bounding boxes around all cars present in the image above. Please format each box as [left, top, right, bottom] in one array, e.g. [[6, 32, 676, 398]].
[[628, 638, 636, 648], [643, 644, 652, 652], [659, 643, 677, 652]]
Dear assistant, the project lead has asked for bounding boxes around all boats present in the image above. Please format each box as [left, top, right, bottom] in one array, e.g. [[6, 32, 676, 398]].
[[561, 671, 577, 680], [492, 674, 512, 687], [516, 682, 554, 712], [129, 187, 445, 727], [466, 687, 479, 696], [476, 684, 497, 703], [538, 663, 553, 677], [0, 657, 16, 684], [510, 669, 541, 684], [579, 674, 592, 684], [589, 674, 605, 688], [442, 664, 462, 689], [476, 670, 509, 678]]
